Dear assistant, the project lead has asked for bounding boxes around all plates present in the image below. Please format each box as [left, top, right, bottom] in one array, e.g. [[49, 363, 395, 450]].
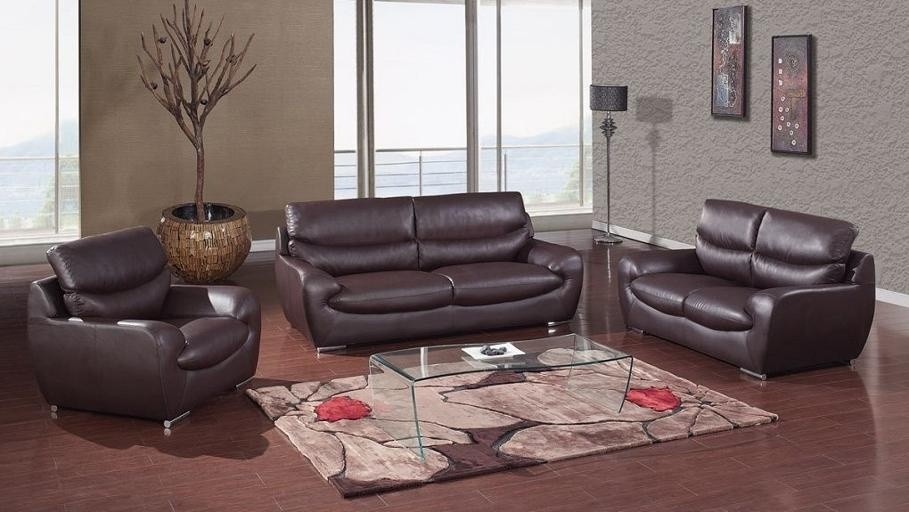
[[461, 342, 527, 361]]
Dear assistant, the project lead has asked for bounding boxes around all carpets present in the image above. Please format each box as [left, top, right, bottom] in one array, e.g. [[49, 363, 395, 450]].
[[245, 348, 778, 501]]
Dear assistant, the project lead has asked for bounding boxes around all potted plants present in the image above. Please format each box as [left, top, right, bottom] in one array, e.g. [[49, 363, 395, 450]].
[[138, 0, 256, 286]]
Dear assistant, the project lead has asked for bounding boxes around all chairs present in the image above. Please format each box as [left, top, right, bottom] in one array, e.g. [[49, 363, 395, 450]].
[[27, 224, 262, 426]]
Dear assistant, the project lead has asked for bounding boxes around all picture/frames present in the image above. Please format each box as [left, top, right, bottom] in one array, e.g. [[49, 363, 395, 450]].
[[770, 34, 812, 155], [711, 6, 746, 119]]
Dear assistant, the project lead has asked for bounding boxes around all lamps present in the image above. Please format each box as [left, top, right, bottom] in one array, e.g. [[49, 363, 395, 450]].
[[588, 84, 628, 245]]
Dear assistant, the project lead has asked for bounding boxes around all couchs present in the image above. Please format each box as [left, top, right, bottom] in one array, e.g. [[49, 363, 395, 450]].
[[273, 193, 583, 352], [616, 198, 877, 379]]
